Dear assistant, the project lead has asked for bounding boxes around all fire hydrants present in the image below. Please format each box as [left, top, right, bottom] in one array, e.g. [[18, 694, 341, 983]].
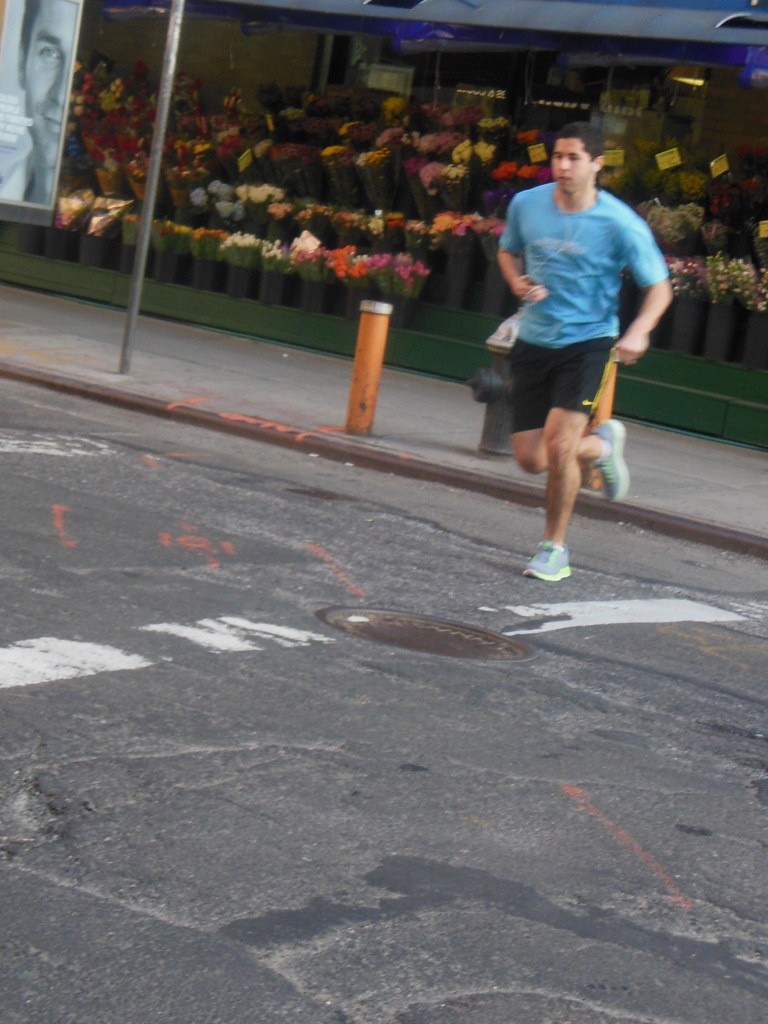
[[466, 354, 519, 463]]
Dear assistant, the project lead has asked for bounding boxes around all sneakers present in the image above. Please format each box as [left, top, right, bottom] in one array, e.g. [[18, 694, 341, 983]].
[[588, 419, 631, 503], [522, 540, 571, 581]]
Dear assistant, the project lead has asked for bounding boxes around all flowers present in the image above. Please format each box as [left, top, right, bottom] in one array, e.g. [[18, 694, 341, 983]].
[[56, 57, 768, 315]]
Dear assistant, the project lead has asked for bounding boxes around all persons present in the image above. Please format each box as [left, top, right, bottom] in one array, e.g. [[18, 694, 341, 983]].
[[0, 0, 78, 205], [496, 122, 672, 583]]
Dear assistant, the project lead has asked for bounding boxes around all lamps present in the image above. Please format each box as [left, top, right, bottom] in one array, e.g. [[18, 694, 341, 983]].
[[668, 64, 706, 86]]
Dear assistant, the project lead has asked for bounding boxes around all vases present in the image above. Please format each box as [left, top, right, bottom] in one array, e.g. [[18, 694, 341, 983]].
[[701, 302, 736, 360], [478, 262, 508, 314], [44, 227, 79, 262], [76, 233, 111, 268], [18, 222, 46, 256], [378, 293, 418, 329], [222, 262, 258, 299], [739, 313, 768, 369], [182, 256, 225, 291], [152, 253, 186, 282], [291, 277, 329, 314], [254, 271, 292, 304], [336, 285, 374, 323], [669, 297, 704, 354], [115, 246, 149, 275], [438, 254, 473, 309]]
